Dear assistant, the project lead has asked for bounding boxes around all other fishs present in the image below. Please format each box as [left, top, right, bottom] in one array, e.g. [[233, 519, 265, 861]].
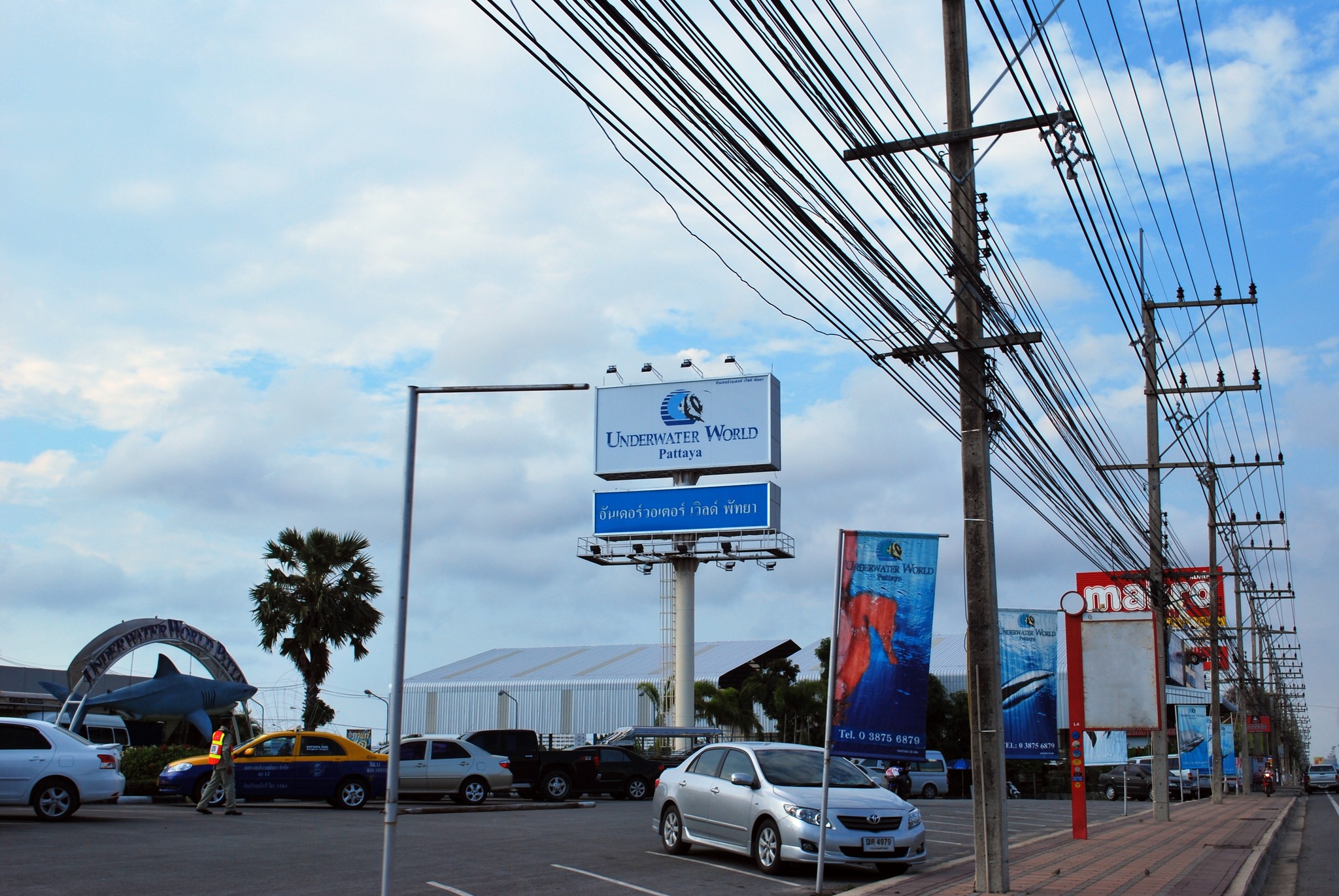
[[1001, 668, 1054, 711], [1179, 729, 1204, 753], [1025, 613, 1038, 628]]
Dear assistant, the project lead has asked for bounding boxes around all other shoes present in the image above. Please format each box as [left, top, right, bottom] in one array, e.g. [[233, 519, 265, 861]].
[[225, 810, 242, 815], [196, 809, 212, 814]]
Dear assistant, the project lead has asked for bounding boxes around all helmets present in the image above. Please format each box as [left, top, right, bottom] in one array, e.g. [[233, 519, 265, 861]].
[[1265, 761, 1271, 765]]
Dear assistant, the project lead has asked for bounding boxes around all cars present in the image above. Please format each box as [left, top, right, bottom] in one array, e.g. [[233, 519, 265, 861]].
[[368, 736, 514, 807], [560, 744, 666, 801], [156, 725, 390, 809], [1097, 763, 1199, 804], [1304, 763, 1339, 794], [667, 743, 716, 762], [0, 718, 127, 823], [652, 741, 929, 876]]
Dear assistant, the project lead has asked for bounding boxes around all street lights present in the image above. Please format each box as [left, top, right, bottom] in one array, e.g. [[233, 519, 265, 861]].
[[364, 690, 389, 745], [272, 724, 283, 731], [497, 688, 518, 730]]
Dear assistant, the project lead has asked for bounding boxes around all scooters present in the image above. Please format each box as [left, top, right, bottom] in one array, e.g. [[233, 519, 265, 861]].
[[1007, 781, 1021, 800], [1257, 769, 1278, 797]]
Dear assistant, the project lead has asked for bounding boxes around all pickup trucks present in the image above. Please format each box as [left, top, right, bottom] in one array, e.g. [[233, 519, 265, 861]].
[[456, 728, 601, 803]]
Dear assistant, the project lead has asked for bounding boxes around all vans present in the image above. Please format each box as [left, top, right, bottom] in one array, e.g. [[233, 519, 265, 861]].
[[1125, 753, 1212, 799], [847, 749, 950, 799], [8, 712, 133, 751], [597, 725, 730, 770]]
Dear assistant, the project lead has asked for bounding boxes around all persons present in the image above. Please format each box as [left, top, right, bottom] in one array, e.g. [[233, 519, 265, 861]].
[[1006, 780, 1010, 798], [881, 759, 914, 801], [195, 710, 244, 816], [1260, 761, 1275, 792]]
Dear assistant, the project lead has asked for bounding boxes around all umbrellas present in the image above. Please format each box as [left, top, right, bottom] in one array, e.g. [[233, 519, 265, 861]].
[[946, 758, 972, 800]]
